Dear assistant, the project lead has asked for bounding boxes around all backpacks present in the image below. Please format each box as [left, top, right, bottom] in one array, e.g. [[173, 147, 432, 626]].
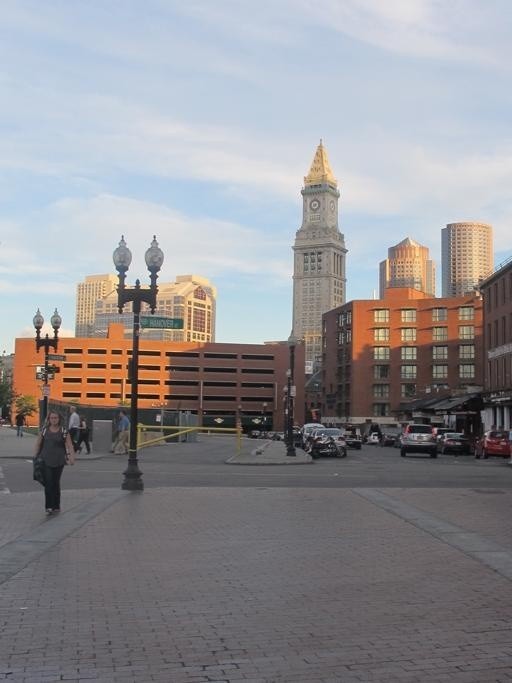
[[32, 455, 45, 486]]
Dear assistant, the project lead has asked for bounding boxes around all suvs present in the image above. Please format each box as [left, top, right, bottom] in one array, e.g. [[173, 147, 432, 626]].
[[401, 424, 437, 457]]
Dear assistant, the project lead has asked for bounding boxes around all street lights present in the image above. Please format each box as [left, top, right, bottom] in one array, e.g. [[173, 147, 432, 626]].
[[238, 403, 243, 424], [283, 367, 291, 445], [33, 306, 63, 424], [263, 402, 268, 435], [286, 329, 298, 457], [113, 233, 163, 492]]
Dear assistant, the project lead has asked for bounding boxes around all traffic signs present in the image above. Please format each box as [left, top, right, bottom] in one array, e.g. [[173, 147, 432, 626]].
[[47, 366, 60, 372], [47, 353, 66, 361], [140, 314, 184, 329]]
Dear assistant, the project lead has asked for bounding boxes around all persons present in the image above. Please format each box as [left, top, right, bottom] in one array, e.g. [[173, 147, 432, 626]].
[[15, 412, 27, 437], [72, 414, 90, 455], [111, 410, 129, 454], [68, 405, 82, 453], [32, 409, 74, 514]]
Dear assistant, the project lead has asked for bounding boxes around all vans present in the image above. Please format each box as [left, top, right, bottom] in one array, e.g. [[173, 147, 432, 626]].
[[432, 427, 455, 451]]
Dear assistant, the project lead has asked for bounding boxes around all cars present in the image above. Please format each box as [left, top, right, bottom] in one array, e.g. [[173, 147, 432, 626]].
[[439, 432, 469, 455], [292, 423, 401, 458], [250, 430, 284, 441], [473, 431, 511, 458]]
